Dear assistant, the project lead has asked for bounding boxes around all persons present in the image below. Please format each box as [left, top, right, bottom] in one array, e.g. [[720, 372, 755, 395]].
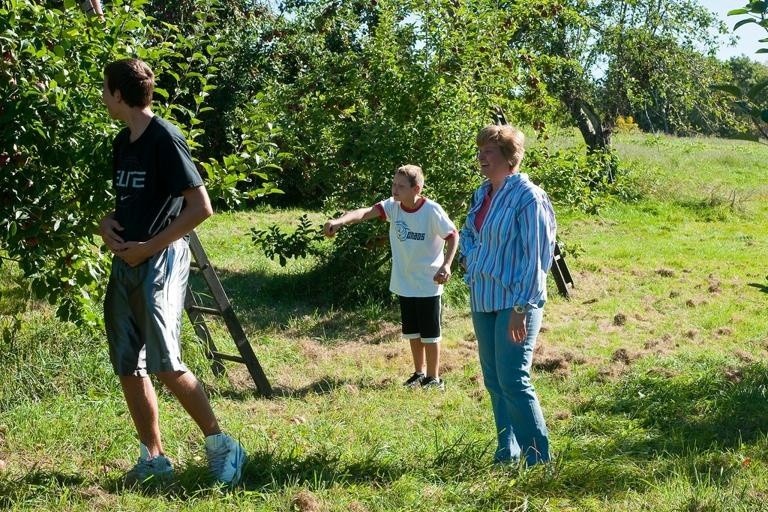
[[98, 58, 243, 484], [325, 163, 462, 387], [462, 125, 559, 466]]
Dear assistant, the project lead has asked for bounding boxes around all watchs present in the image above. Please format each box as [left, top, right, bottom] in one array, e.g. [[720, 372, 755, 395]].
[[513, 305, 526, 315]]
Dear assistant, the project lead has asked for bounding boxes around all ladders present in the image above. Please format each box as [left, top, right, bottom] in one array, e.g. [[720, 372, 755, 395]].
[[439, 0, 574, 303], [78, 0, 274, 400]]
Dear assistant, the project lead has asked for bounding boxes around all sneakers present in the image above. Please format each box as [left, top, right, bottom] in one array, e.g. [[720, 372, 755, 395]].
[[125, 443, 175, 482], [404, 371, 426, 386], [205, 431, 247, 489], [421, 376, 445, 390]]
